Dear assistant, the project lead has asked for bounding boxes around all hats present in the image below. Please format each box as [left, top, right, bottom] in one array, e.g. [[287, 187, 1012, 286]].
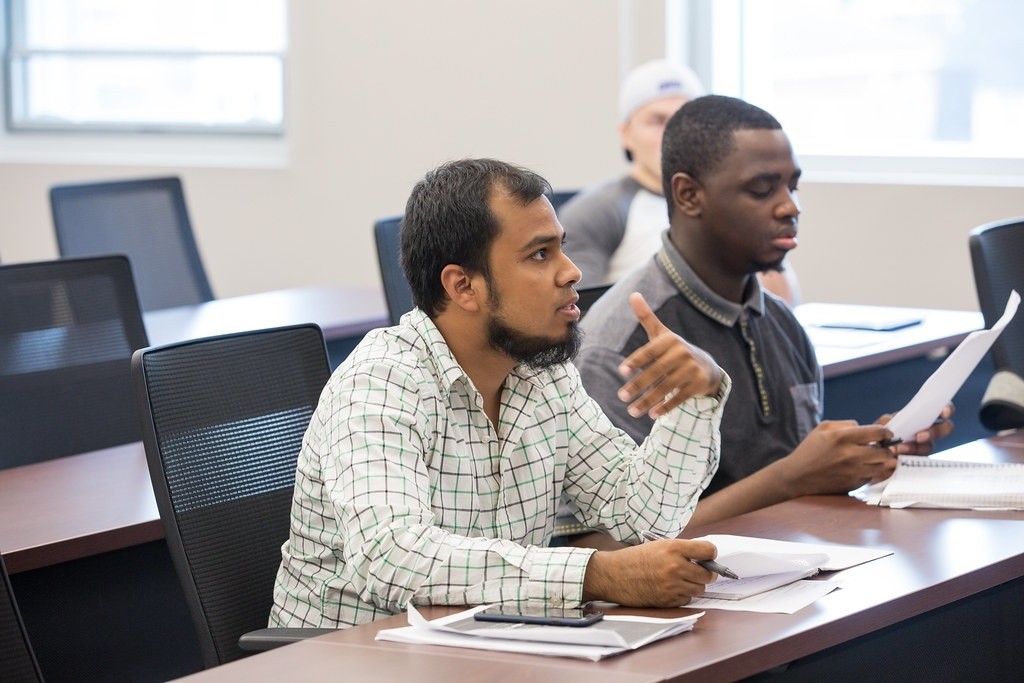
[[619, 59, 705, 165]]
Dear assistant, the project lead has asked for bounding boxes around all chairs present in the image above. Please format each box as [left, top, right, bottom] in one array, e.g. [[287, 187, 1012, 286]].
[[129, 322, 345, 670], [373, 215, 415, 327], [968, 216, 1024, 383], [0, 255, 152, 472], [50, 175, 216, 312]]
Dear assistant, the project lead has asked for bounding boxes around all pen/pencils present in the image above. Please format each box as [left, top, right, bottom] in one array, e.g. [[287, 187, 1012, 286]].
[[642, 530, 742, 581], [874, 437, 904, 450]]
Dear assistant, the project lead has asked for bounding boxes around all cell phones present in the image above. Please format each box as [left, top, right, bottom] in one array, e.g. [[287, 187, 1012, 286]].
[[473, 603, 603, 626]]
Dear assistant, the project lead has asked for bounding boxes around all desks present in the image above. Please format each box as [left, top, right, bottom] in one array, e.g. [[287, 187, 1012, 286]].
[[0, 300, 990, 683], [166, 428, 1024, 683], [0, 289, 391, 472]]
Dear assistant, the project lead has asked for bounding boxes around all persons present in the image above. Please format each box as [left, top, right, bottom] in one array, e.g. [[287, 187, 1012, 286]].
[[553, 61, 803, 308], [573, 96, 956, 530], [268, 157, 734, 628]]
